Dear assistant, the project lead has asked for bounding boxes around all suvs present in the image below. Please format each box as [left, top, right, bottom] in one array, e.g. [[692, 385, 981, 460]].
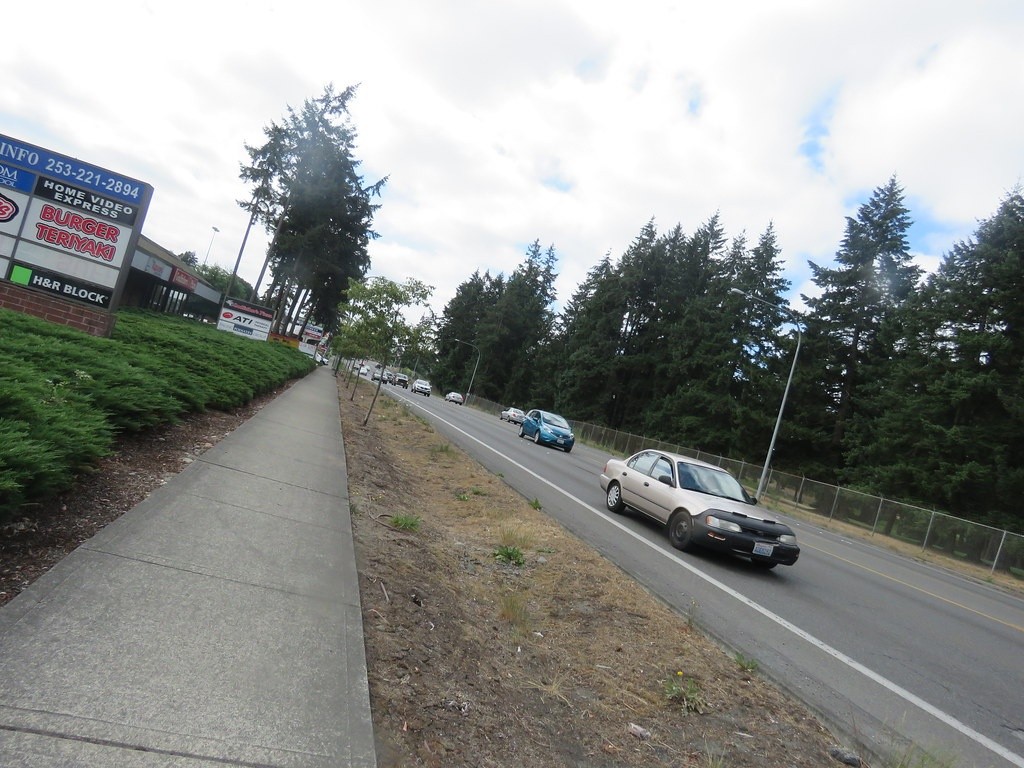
[[411, 379, 433, 397]]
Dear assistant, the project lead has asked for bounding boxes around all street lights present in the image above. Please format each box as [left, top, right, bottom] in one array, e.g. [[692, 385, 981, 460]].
[[454, 338, 481, 406], [204, 225, 219, 265], [730, 288, 803, 503]]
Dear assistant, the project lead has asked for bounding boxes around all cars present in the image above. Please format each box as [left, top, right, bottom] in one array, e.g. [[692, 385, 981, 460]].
[[315, 353, 329, 366], [517, 408, 574, 453], [371, 370, 409, 389], [355, 361, 370, 376], [445, 391, 463, 405], [598, 449, 801, 572], [499, 407, 525, 424]]
